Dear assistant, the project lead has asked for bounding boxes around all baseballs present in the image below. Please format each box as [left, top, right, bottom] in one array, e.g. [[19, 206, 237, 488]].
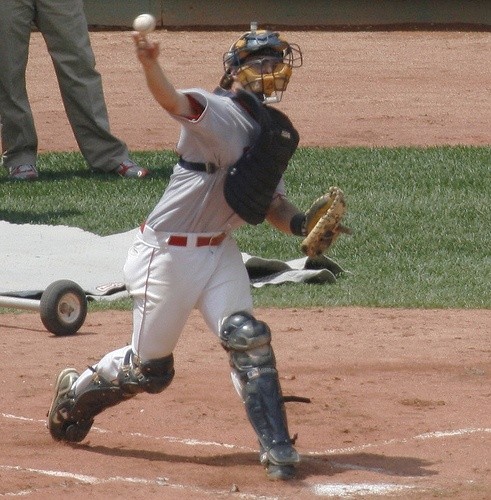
[[133, 14, 156, 34]]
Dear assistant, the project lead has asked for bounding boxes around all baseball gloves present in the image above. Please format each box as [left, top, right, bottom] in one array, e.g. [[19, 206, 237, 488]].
[[299, 186, 353, 258]]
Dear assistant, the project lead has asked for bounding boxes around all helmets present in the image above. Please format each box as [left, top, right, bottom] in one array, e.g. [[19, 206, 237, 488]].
[[220, 29, 303, 108]]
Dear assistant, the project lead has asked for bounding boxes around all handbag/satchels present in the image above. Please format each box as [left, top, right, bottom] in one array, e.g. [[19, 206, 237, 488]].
[[179, 87, 300, 224]]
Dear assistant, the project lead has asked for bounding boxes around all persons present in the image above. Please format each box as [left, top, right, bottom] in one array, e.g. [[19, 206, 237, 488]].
[[0, 0, 148, 181], [46, 22, 346, 479]]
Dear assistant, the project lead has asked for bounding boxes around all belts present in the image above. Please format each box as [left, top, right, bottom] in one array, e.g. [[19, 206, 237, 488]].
[[141, 222, 227, 247]]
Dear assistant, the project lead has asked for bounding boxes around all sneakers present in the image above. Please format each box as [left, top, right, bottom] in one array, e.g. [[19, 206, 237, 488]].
[[261, 440, 294, 479], [115, 159, 147, 179], [48, 368, 78, 442], [8, 165, 38, 179]]
